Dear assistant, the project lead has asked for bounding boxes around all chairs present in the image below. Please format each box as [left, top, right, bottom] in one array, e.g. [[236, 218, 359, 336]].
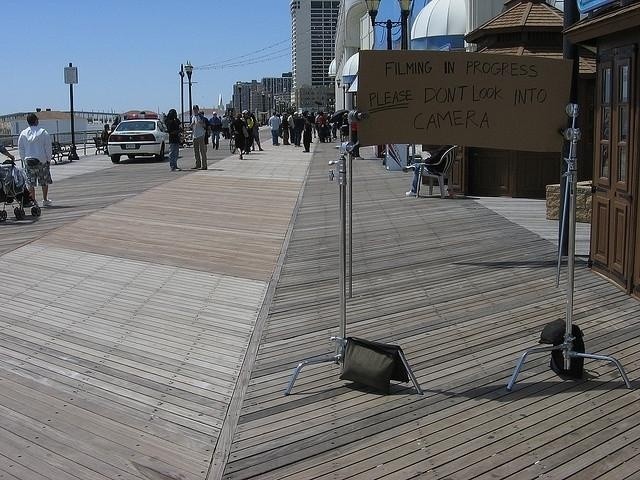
[[413, 145, 457, 199]]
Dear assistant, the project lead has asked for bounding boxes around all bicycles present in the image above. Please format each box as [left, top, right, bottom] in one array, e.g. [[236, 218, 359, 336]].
[[228, 133, 237, 154]]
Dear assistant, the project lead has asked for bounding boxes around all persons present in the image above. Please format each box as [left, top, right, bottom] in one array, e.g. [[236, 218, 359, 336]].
[[101, 124, 112, 155], [188, 105, 263, 170], [0, 145, 15, 162], [403, 145, 454, 197], [18, 113, 53, 207], [2, 159, 24, 190], [164, 108, 183, 172], [269, 110, 348, 152]]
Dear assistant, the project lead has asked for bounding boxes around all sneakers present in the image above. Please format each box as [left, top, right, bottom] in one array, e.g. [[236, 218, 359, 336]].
[[403, 165, 415, 173], [406, 190, 417, 196], [43, 200, 52, 205]]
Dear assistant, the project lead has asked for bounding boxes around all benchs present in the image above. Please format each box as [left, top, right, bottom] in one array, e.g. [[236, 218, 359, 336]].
[[93, 136, 108, 155], [50, 141, 74, 165]]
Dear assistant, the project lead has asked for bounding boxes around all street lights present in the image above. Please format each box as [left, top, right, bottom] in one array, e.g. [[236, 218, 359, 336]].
[[184, 61, 194, 125]]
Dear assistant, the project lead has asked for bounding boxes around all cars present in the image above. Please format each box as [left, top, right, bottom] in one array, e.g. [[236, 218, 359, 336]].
[[105, 117, 170, 164]]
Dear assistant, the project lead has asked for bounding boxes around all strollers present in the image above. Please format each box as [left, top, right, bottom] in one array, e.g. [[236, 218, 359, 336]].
[[0, 159, 42, 222]]
